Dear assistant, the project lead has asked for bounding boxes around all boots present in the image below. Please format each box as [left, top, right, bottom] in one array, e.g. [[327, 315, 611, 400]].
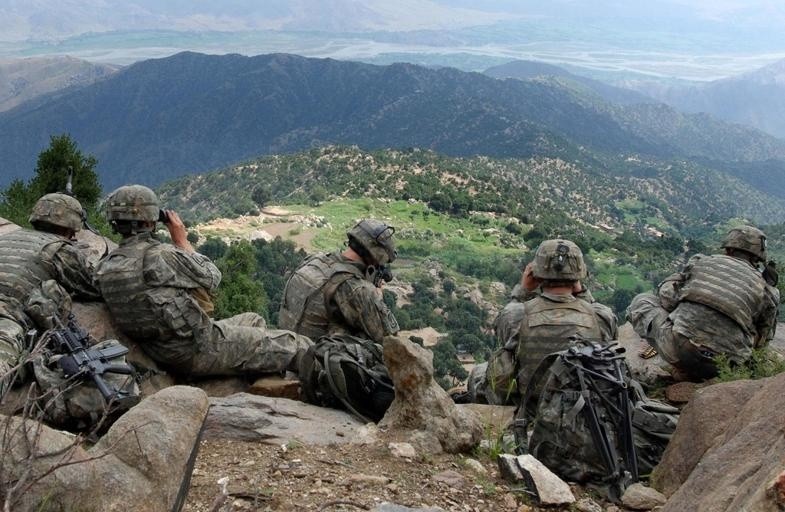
[[247, 376, 301, 400], [664, 377, 717, 403]]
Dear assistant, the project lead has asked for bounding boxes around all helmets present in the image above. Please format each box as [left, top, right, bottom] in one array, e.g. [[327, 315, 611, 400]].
[[28, 192, 84, 232], [720, 224, 767, 260], [105, 183, 160, 223], [346, 218, 398, 266], [532, 239, 587, 281]]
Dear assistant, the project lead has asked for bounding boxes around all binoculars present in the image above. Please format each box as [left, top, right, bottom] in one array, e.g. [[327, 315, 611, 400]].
[[157, 210, 170, 224]]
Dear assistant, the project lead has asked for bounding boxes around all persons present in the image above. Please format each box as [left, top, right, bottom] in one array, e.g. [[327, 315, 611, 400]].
[[625, 224, 780, 402], [97, 184, 314, 383], [0, 191, 103, 408], [468, 238, 619, 408], [278, 218, 400, 342]]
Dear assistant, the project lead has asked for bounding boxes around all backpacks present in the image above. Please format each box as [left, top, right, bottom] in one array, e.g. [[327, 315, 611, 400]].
[[512, 339, 641, 484], [31, 338, 142, 428], [629, 380, 681, 476], [301, 330, 394, 423]]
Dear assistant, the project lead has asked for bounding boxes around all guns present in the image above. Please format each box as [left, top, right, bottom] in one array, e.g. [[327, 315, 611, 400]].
[[52, 315, 136, 406]]
